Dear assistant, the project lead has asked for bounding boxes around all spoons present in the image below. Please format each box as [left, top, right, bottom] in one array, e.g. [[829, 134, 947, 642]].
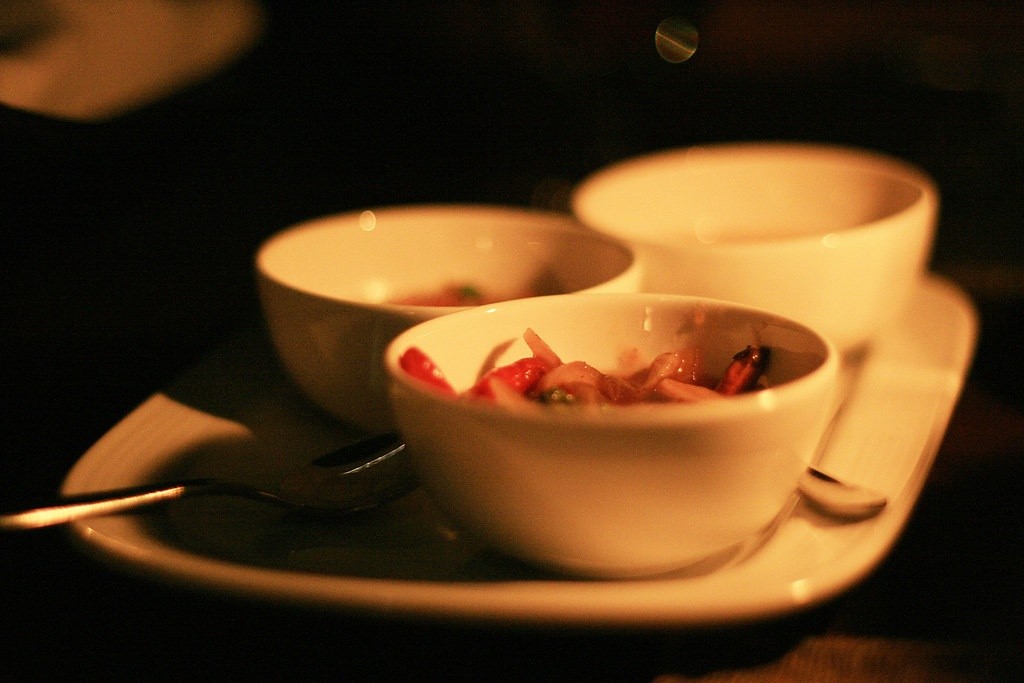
[[0, 433, 420, 536]]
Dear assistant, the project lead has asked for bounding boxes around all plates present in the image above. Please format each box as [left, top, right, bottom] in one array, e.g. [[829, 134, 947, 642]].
[[57, 271, 980, 623]]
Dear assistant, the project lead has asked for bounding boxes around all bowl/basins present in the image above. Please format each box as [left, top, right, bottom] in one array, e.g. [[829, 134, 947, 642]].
[[570, 142, 935, 352], [251, 204, 639, 437], [384, 294, 839, 576]]
